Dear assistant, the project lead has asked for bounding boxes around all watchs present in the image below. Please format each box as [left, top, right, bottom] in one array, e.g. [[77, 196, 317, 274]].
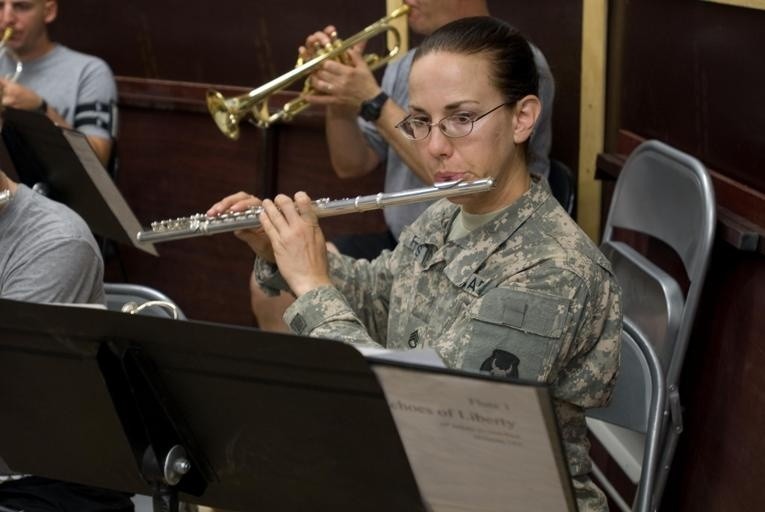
[[355, 91, 390, 123]]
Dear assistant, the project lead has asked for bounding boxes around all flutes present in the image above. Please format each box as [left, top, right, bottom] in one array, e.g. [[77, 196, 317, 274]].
[[138, 175, 497, 244]]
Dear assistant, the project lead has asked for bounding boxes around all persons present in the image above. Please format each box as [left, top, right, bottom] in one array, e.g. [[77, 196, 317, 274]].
[[1, 169, 108, 305], [204, 15, 627, 509], [0, 1, 124, 170], [295, 0, 558, 264]]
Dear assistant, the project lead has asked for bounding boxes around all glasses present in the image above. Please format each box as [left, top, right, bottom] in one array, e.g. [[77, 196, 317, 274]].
[[395, 102, 512, 140]]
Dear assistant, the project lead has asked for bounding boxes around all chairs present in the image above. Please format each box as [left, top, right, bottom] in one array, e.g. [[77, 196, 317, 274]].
[[581, 316, 667, 511], [596, 138, 715, 512], [102, 282, 189, 321]]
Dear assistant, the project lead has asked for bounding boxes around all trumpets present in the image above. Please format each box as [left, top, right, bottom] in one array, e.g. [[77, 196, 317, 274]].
[[0, 26, 24, 83], [205, 1, 410, 141]]
